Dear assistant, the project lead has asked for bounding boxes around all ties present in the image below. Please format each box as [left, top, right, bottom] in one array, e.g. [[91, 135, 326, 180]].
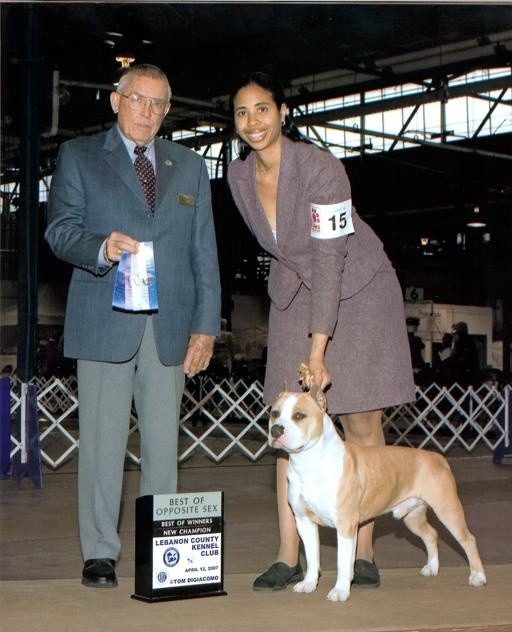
[[133, 146, 155, 217]]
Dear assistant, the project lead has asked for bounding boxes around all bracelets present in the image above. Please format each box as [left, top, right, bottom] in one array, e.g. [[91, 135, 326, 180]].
[[103, 245, 114, 268]]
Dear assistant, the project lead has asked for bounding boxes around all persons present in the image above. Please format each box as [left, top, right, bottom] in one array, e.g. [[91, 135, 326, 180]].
[[451, 322, 475, 353], [441, 334, 453, 361], [228, 75, 416, 591], [406, 317, 425, 368], [45, 66, 221, 587]]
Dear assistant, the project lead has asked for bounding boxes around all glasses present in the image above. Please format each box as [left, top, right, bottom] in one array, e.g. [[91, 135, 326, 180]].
[[121, 92, 171, 117]]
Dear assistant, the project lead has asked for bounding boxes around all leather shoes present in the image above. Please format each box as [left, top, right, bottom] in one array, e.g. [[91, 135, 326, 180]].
[[352, 557, 379, 588], [253, 562, 304, 591], [82, 559, 118, 588]]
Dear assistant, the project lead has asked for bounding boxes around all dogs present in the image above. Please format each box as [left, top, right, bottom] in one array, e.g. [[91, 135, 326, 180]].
[[267, 379, 487, 602]]
